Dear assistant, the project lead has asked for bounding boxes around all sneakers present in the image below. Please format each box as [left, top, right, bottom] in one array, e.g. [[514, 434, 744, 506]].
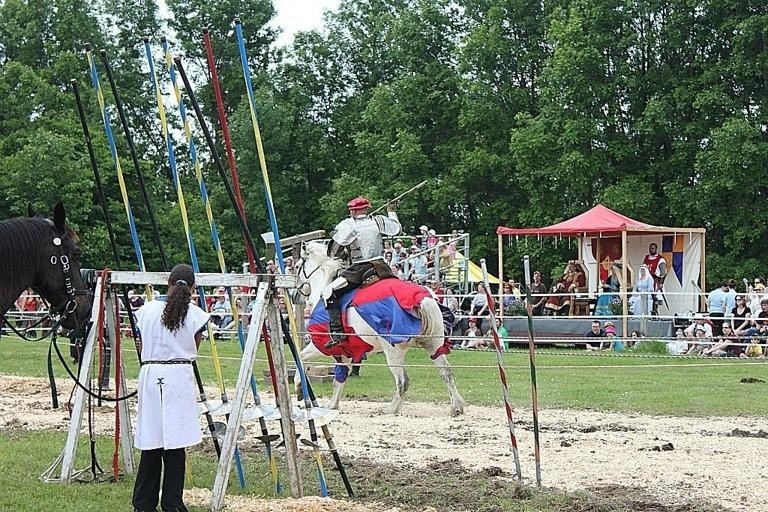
[[696, 325, 706, 333]]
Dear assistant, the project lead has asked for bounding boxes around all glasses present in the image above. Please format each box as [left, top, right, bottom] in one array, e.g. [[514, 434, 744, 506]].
[[736, 299, 741, 301], [754, 281, 760, 284]]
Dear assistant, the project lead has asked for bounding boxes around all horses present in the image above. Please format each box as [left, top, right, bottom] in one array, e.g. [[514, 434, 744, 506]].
[[0, 202, 91, 331], [293, 239, 466, 417]]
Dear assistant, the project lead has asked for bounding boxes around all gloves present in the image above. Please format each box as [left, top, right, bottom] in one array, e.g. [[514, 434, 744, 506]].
[[387, 199, 398, 212]]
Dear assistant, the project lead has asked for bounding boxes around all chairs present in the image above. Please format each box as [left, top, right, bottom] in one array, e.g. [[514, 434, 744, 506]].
[[597, 259, 636, 301], [549, 260, 589, 319]]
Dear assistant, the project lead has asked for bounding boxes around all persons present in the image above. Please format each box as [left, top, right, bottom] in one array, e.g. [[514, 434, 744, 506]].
[[321, 196, 403, 350], [16, 224, 767, 378], [130, 262, 211, 511]]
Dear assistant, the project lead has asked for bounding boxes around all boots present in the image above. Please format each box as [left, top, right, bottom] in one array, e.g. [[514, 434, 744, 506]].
[[324, 309, 346, 347]]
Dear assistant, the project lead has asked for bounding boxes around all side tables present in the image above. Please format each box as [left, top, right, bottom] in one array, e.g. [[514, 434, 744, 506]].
[[575, 299, 597, 316]]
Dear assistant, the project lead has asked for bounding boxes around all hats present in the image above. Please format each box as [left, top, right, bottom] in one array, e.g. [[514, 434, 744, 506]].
[[692, 313, 704, 320], [469, 318, 477, 322], [395, 225, 436, 253], [347, 198, 372, 210], [604, 322, 615, 333]]
